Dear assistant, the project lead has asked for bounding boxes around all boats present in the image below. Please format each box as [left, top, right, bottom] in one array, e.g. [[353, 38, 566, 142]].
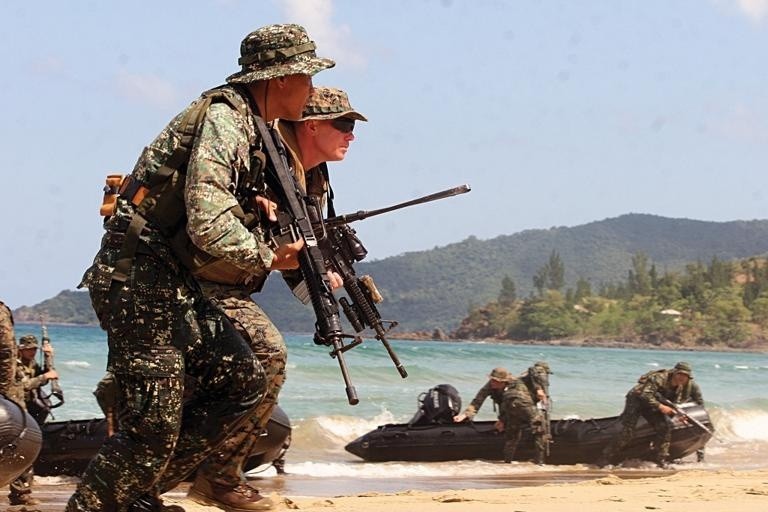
[[32, 391, 292, 477], [0, 396, 43, 489], [344, 384, 713, 463]]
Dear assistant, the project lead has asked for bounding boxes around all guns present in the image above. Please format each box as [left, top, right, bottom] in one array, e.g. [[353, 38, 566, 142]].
[[239, 127, 363, 406], [308, 172, 408, 380], [653, 391, 719, 440], [540, 381, 554, 458], [39, 323, 64, 401]]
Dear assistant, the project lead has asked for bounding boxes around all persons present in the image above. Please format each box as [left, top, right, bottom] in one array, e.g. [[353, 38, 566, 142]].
[[452, 367, 522, 432], [9, 332, 65, 505], [598, 361, 692, 478], [63, 24, 336, 512], [499, 361, 554, 468], [190, 87, 369, 512], [0, 297, 42, 488], [683, 377, 714, 463]]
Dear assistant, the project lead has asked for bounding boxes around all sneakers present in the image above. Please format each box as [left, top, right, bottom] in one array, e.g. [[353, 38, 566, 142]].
[[187, 468, 273, 512]]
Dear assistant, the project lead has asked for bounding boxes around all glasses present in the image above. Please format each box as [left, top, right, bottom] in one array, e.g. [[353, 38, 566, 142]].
[[331, 117, 355, 132]]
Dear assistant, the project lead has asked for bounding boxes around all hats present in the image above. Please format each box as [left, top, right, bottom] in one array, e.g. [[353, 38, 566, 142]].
[[488, 367, 511, 383], [671, 361, 693, 379], [279, 87, 367, 121], [225, 24, 336, 84], [18, 335, 42, 350], [535, 361, 554, 374]]
